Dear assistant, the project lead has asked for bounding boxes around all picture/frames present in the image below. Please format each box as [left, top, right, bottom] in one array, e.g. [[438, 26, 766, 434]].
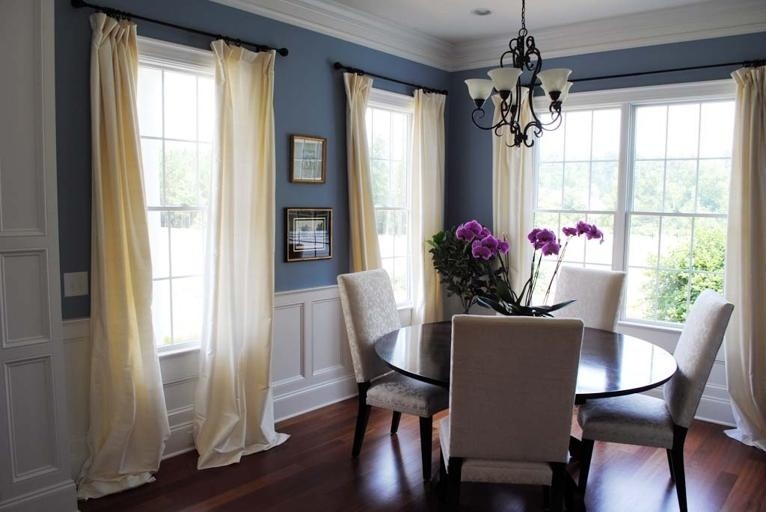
[[281, 131, 333, 263]]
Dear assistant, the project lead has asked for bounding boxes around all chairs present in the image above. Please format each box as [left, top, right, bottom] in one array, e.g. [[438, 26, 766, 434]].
[[436, 313, 586, 510], [577, 288, 735, 512], [336, 267, 448, 481], [551, 262, 627, 331]]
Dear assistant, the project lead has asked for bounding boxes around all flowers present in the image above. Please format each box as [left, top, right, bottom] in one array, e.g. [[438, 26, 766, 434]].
[[454, 211, 602, 317]]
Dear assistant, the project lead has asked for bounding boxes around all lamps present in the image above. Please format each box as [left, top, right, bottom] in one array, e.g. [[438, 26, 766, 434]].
[[459, 2, 575, 148]]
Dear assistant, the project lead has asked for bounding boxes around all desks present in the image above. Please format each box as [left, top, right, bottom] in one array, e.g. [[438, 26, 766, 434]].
[[372, 319, 679, 399]]
